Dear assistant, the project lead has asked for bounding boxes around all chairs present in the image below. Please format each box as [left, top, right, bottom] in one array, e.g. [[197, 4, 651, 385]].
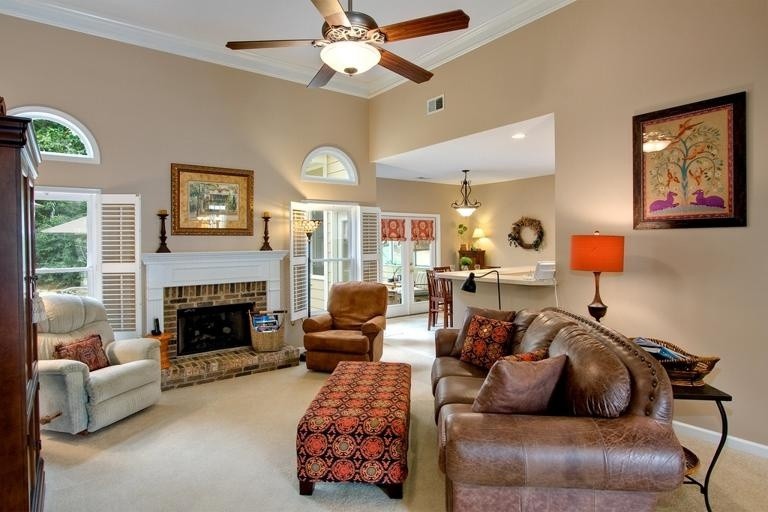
[[33, 290, 163, 435], [302, 278, 387, 374], [425, 265, 452, 331]]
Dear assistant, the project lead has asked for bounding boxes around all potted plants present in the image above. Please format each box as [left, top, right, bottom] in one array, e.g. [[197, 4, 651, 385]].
[[457, 223, 468, 251], [461, 257, 472, 270]]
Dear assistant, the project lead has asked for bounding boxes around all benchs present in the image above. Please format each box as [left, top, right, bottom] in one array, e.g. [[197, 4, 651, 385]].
[[297, 361, 411, 499]]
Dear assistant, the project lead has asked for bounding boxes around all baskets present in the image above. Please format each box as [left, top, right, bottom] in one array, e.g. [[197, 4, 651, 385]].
[[681, 445, 701, 477], [249, 311, 284, 353], [628, 337, 722, 388]]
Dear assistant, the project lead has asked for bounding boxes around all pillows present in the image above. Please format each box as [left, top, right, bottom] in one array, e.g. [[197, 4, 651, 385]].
[[471, 354, 568, 411], [496, 346, 551, 363], [53, 334, 110, 371], [451, 303, 516, 358], [460, 316, 515, 373]]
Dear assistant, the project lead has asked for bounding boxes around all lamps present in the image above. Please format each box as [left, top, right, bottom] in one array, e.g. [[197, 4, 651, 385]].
[[460, 270, 503, 309], [640, 130, 675, 153], [451, 169, 482, 218], [297, 218, 322, 317], [322, 42, 384, 76], [571, 233, 624, 323]]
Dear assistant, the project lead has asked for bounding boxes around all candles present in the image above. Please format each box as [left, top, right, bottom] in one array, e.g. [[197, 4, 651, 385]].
[[159, 209, 167, 214], [264, 211, 271, 217]]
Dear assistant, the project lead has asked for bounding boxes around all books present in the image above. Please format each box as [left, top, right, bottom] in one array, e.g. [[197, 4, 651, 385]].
[[632, 336, 708, 370], [252, 312, 279, 333]]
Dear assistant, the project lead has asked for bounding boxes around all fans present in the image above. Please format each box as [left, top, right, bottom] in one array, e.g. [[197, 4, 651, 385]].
[[225, 0, 470, 92]]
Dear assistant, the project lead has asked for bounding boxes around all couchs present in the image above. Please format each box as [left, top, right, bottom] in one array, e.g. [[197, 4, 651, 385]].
[[429, 307, 686, 511]]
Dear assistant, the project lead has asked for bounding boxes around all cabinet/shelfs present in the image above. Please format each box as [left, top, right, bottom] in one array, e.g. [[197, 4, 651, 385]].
[[459, 250, 485, 266], [0, 115, 46, 512]]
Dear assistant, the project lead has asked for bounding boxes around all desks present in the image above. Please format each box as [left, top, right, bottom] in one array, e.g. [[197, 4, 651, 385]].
[[669, 381, 731, 510]]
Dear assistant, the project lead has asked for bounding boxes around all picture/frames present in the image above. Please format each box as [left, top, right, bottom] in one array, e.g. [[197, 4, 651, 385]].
[[169, 162, 255, 236], [630, 90, 750, 231]]
